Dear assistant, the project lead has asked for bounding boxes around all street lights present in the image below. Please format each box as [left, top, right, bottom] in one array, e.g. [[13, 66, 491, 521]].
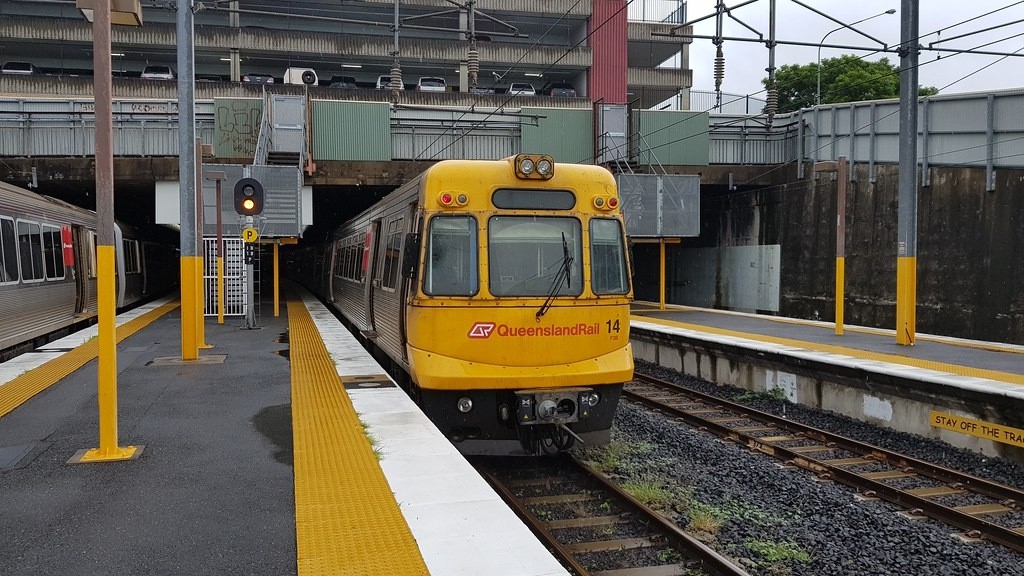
[[814, 155, 847, 335], [75, 0, 144, 461], [818, 9, 896, 105], [206, 171, 227, 325], [196, 137, 215, 349]]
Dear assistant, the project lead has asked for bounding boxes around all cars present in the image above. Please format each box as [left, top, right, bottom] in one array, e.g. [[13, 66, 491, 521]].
[[546, 84, 577, 98], [1, 61, 40, 76], [377, 75, 404, 91], [242, 72, 277, 85], [437, 89, 438, 90], [140, 65, 177, 80], [331, 77, 356, 88], [506, 83, 536, 95]]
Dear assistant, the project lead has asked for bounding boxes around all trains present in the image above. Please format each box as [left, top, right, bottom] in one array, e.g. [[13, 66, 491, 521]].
[[287, 153, 634, 457], [0, 181, 181, 363]]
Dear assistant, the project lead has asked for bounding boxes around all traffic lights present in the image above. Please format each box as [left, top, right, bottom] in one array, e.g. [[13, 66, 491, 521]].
[[234, 178, 264, 215]]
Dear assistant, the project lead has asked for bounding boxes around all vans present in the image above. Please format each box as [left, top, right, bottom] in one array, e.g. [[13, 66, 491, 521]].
[[415, 78, 448, 92]]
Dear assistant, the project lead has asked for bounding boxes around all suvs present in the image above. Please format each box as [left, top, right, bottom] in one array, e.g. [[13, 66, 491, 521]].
[[284, 68, 319, 85]]
